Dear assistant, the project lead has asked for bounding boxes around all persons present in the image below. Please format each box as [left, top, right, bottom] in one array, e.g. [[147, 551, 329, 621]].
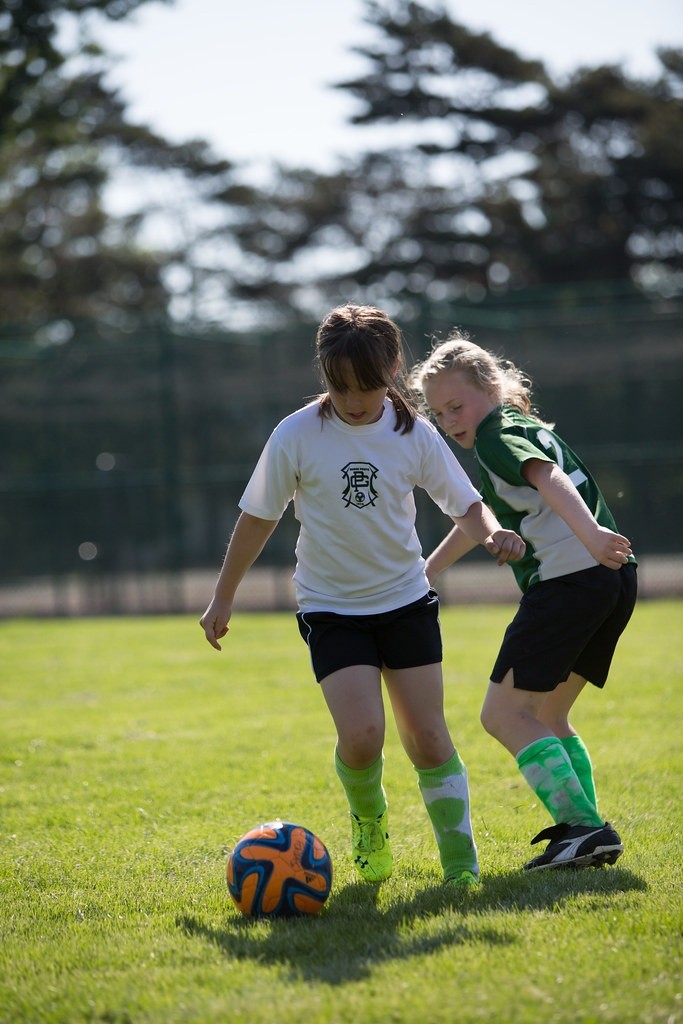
[[409, 338, 640, 868], [200, 304, 526, 884]]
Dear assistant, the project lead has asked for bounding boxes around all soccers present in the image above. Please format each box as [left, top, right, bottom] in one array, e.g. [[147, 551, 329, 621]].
[[222, 818, 336, 923]]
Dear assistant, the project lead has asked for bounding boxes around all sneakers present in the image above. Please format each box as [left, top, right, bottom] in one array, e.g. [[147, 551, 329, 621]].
[[443, 871, 484, 900], [521, 821, 624, 873], [349, 799, 393, 882]]
